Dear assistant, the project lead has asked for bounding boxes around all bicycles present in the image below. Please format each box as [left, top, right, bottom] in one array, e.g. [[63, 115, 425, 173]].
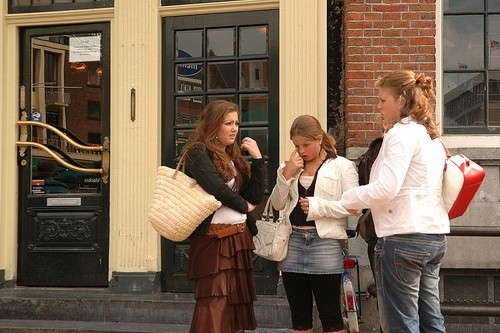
[[341, 229, 370, 333]]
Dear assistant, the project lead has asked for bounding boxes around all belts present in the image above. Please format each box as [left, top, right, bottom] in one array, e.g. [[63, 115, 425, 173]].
[[205, 221, 247, 240]]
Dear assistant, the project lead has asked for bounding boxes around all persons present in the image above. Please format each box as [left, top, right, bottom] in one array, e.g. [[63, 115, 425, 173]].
[[340, 70, 450, 333], [185, 100, 265, 333], [358, 115, 394, 311], [269, 115, 361, 333]]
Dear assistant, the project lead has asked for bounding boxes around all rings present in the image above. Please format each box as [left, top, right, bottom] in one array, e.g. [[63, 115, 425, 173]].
[[306, 207, 309, 211]]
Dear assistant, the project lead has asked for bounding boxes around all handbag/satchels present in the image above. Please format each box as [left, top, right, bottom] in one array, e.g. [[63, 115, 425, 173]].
[[439, 138, 486, 221], [252, 194, 291, 262], [356, 214, 369, 243], [148, 142, 222, 242]]
